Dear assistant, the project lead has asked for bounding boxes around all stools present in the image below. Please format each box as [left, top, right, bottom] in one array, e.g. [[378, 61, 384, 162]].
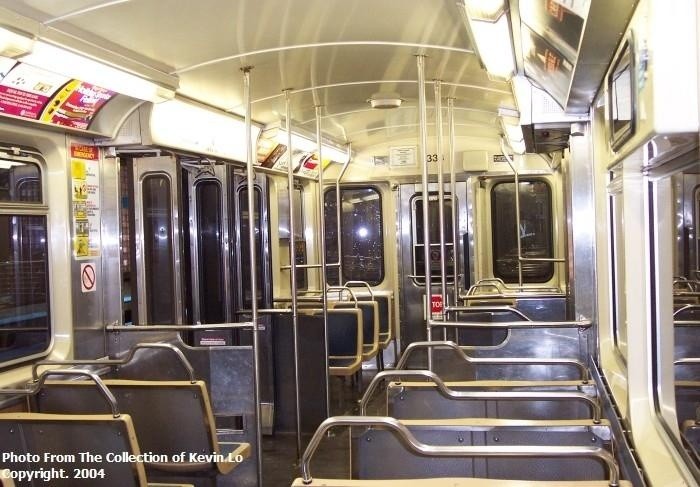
[[668, 268, 700, 462]]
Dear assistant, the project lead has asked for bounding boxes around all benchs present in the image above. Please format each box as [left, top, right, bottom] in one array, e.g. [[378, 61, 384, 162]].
[[1, 277, 636, 487]]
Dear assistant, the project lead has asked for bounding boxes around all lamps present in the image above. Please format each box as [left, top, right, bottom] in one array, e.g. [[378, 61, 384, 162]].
[[459, 0, 518, 85], [499, 104, 529, 158], [1, 24, 178, 107], [260, 118, 357, 166]]
